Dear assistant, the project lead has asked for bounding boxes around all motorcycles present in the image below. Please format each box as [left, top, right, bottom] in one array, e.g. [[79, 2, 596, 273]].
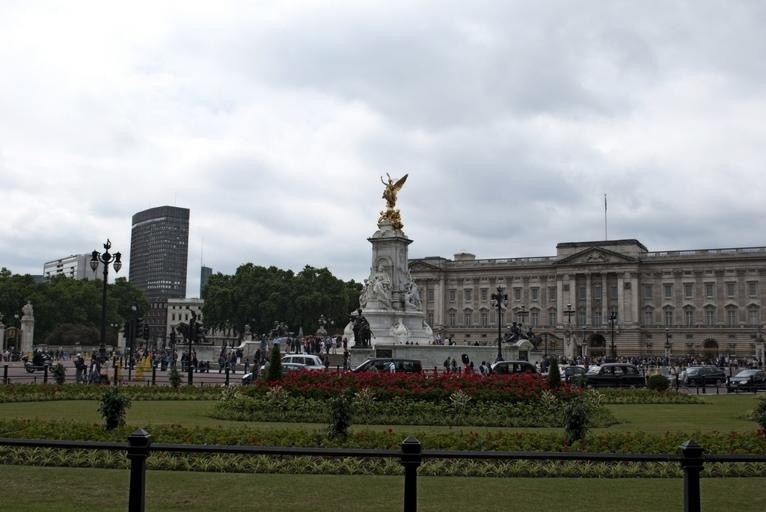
[[21, 353, 57, 374]]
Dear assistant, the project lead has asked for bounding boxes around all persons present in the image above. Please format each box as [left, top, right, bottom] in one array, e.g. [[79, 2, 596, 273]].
[[535, 352, 763, 376], [52, 363, 66, 382], [479, 360, 489, 376], [361, 263, 423, 311], [199, 360, 210, 373], [443, 356, 451, 374], [5, 346, 66, 362], [219, 333, 339, 381], [503, 320, 522, 345], [526, 326, 544, 351], [451, 358, 458, 373], [73, 344, 145, 382], [487, 362, 493, 374], [344, 306, 371, 345], [358, 319, 376, 348], [153, 348, 198, 373]]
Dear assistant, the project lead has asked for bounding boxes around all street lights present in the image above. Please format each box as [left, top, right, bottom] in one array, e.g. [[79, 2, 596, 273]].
[[491, 286, 508, 362], [564, 303, 576, 324], [663, 327, 672, 366], [608, 308, 617, 357], [90, 238, 122, 364]]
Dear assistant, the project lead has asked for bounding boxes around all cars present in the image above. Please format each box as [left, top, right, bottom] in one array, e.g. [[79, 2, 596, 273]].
[[559, 364, 645, 387], [241, 354, 326, 385], [726, 369, 766, 392], [488, 361, 537, 375], [681, 365, 726, 387], [344, 357, 422, 375]]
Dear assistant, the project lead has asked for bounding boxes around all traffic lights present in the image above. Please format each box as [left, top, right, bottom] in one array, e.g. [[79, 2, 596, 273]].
[[121, 321, 130, 339], [144, 326, 149, 340], [134, 318, 143, 339]]
